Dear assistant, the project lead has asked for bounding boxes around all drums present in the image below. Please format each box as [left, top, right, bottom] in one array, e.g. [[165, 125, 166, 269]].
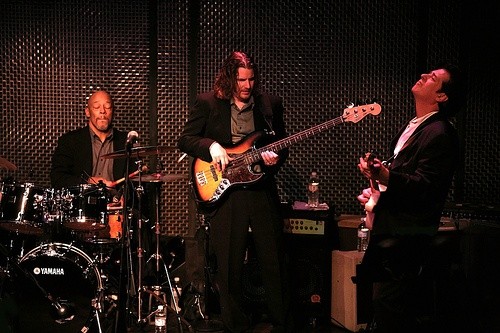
[[81, 207, 131, 244], [22, 243, 101, 333], [60, 184, 108, 230], [0, 183, 56, 234]]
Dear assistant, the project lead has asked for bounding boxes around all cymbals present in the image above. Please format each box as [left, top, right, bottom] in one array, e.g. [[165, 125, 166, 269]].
[[100, 145, 178, 158], [0, 156, 17, 170], [131, 173, 188, 183]]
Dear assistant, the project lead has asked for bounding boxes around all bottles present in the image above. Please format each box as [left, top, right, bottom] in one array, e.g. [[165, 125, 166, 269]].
[[357, 217, 370, 253], [307, 172, 320, 208], [155, 306, 167, 333]]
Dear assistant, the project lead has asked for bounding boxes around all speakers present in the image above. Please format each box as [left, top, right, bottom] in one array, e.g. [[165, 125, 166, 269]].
[[240, 204, 338, 326]]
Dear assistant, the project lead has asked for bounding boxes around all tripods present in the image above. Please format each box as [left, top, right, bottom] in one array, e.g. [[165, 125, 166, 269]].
[[103, 153, 193, 333]]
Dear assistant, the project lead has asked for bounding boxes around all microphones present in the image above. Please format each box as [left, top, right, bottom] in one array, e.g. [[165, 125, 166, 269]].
[[125, 130, 139, 152]]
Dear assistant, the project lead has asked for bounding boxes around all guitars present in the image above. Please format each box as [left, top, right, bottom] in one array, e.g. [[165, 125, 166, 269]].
[[359, 152, 391, 230], [190, 101, 382, 206]]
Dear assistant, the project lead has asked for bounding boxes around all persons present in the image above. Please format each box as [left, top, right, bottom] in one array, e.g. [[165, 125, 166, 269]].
[[356, 68, 468, 333], [51, 91, 157, 258], [177, 51, 294, 333]]
